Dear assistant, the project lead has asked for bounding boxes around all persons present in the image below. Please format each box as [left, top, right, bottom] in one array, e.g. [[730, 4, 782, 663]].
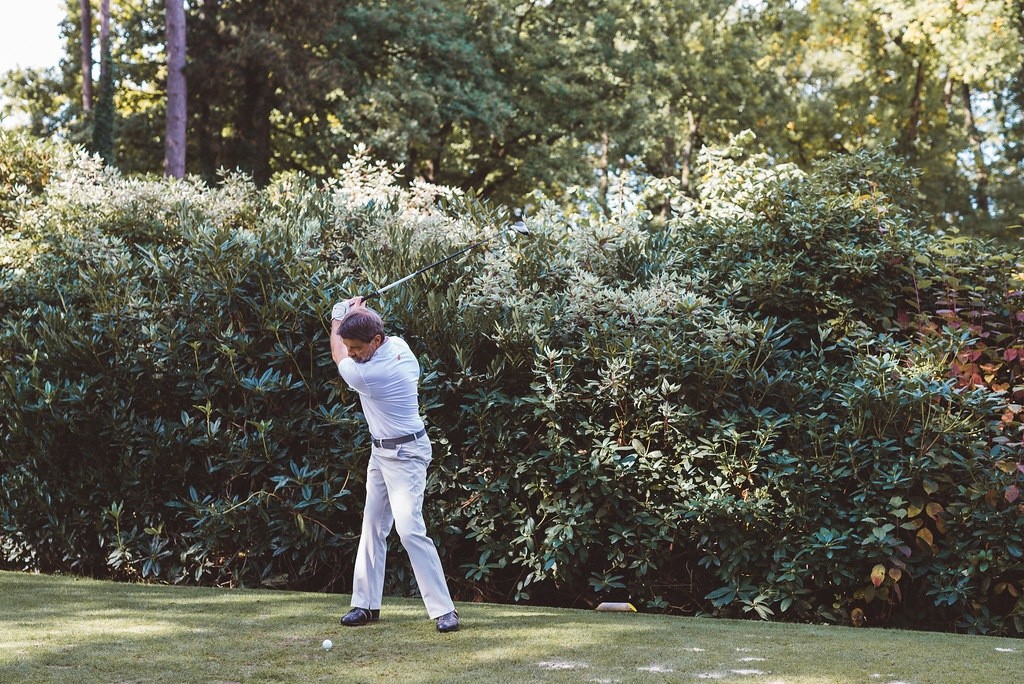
[[329, 296, 459, 633]]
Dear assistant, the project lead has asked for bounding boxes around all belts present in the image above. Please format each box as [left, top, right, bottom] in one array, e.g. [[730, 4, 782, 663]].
[[370, 428, 426, 449]]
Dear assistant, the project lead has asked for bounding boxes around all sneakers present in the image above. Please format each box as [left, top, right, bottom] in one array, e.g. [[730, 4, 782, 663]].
[[436, 609, 459, 632], [341, 606, 380, 626]]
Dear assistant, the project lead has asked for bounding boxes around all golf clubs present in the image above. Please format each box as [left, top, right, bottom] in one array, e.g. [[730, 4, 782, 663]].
[[351, 221, 530, 305]]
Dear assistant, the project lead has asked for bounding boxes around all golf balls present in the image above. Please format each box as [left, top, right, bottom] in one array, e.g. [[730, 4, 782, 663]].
[[322, 639, 333, 649]]
